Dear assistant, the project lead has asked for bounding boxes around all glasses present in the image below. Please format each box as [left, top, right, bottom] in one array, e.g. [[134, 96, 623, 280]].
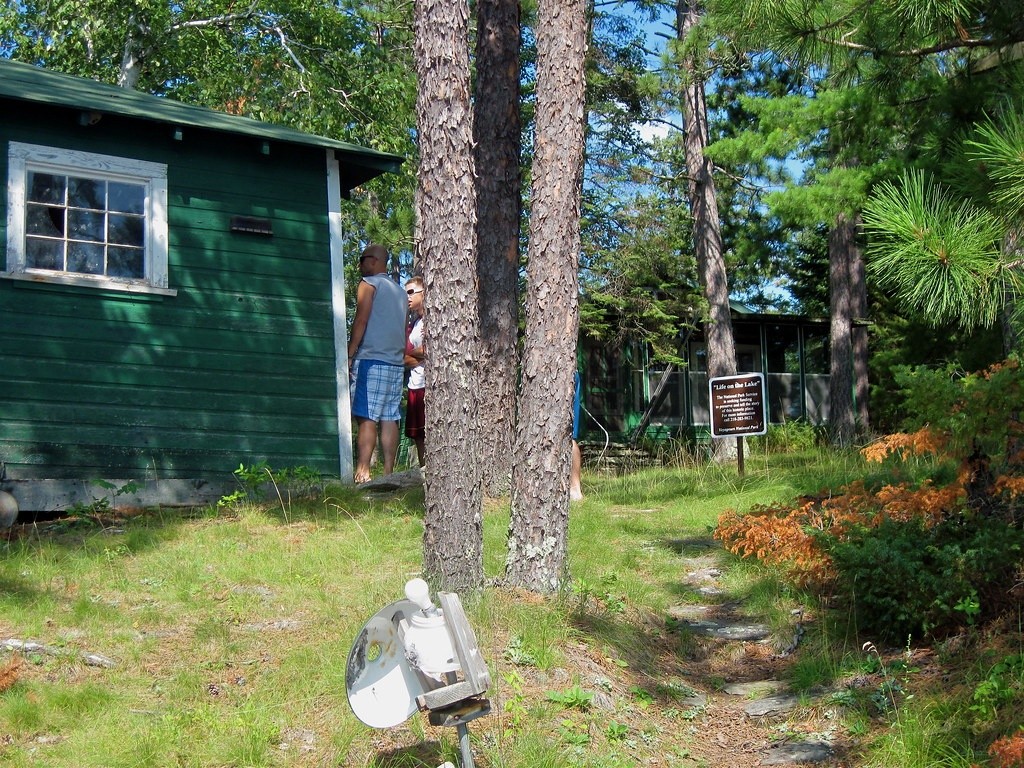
[[360, 255, 378, 264], [407, 289, 424, 296]]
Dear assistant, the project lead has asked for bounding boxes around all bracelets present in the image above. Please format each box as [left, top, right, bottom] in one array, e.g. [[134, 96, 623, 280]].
[[348, 356, 353, 359]]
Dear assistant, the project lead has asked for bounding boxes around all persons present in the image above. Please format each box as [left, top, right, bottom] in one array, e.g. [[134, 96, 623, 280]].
[[348, 246, 409, 483], [571, 356, 582, 499], [405, 276, 428, 502]]
